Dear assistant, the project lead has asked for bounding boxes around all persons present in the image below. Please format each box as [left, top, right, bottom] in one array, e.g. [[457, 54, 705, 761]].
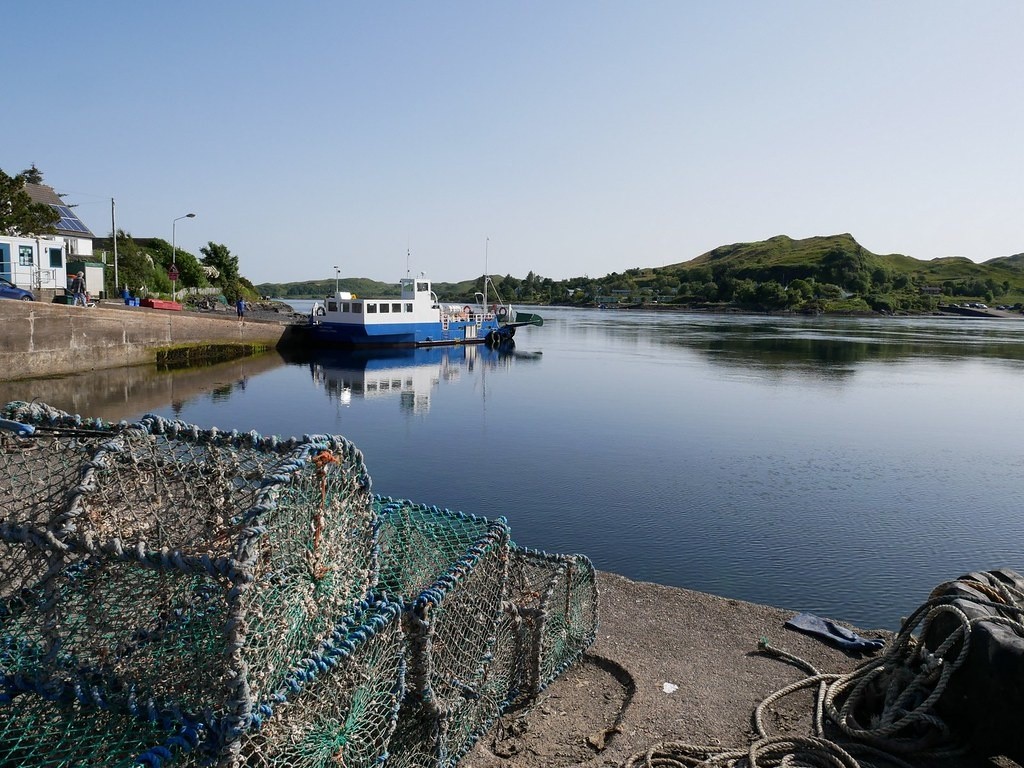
[[71, 271, 87, 308], [237, 297, 251, 321]]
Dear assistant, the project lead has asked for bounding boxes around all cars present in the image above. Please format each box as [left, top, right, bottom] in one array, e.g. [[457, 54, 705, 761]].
[[937, 301, 1014, 310], [0, 276, 36, 301]]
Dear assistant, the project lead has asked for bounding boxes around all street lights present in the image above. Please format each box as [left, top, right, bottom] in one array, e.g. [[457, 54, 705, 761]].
[[173, 213, 196, 303]]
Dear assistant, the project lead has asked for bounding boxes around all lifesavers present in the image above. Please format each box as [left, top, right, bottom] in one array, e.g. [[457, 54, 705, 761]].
[[317, 306, 325, 316], [490, 331, 500, 343], [499, 307, 507, 316], [463, 305, 472, 313]]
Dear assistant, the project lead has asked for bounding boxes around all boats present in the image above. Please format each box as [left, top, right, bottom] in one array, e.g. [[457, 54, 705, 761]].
[[300, 235, 544, 347]]
